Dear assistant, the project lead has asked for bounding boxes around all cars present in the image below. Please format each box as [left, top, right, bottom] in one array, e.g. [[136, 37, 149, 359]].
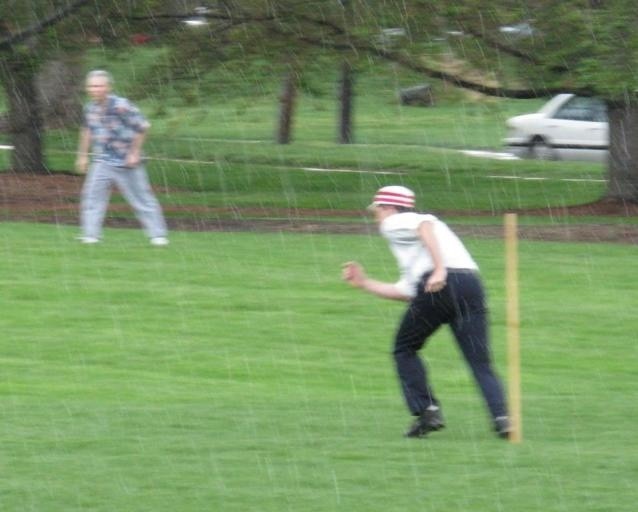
[[504, 87, 615, 166]]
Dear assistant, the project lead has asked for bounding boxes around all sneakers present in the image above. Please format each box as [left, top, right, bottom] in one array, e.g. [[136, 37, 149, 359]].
[[73, 235, 168, 245], [402, 406, 446, 440], [492, 414, 511, 438]]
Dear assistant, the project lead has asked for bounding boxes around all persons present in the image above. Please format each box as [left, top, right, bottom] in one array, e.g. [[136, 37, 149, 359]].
[[75, 70, 170, 248], [338, 183, 513, 443]]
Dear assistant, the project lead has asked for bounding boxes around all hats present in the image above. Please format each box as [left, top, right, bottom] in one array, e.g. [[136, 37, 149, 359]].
[[364, 184, 418, 211]]
